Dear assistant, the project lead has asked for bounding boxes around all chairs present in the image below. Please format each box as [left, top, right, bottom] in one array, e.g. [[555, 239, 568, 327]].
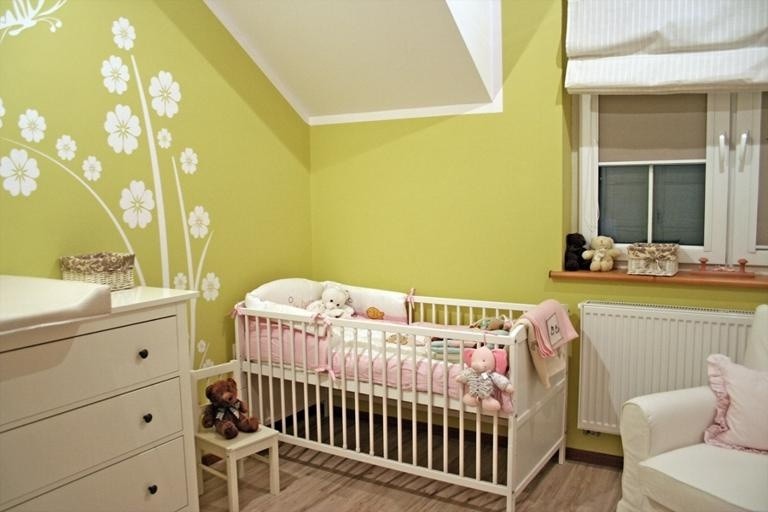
[[190, 360, 281, 511], [616, 303, 767, 510]]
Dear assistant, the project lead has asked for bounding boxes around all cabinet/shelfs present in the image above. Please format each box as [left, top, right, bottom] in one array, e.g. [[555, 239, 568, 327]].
[[0, 275, 202, 512]]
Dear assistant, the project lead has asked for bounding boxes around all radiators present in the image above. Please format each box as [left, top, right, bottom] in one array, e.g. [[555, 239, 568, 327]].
[[576, 299, 754, 438]]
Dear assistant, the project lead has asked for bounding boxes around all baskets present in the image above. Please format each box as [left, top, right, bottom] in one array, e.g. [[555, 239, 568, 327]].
[[626, 242, 678, 276], [60, 252, 134, 291]]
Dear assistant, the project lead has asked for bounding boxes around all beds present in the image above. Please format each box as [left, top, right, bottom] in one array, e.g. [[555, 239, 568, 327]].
[[232, 277, 580, 511]]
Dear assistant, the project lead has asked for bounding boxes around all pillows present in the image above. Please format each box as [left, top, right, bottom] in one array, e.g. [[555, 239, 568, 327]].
[[704, 349, 766, 455]]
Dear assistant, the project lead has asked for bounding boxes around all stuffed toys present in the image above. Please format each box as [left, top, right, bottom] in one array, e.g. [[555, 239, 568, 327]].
[[202, 377, 258, 439], [565, 233, 591, 271], [455, 346, 514, 412], [306, 281, 355, 319], [581, 236, 621, 272]]
